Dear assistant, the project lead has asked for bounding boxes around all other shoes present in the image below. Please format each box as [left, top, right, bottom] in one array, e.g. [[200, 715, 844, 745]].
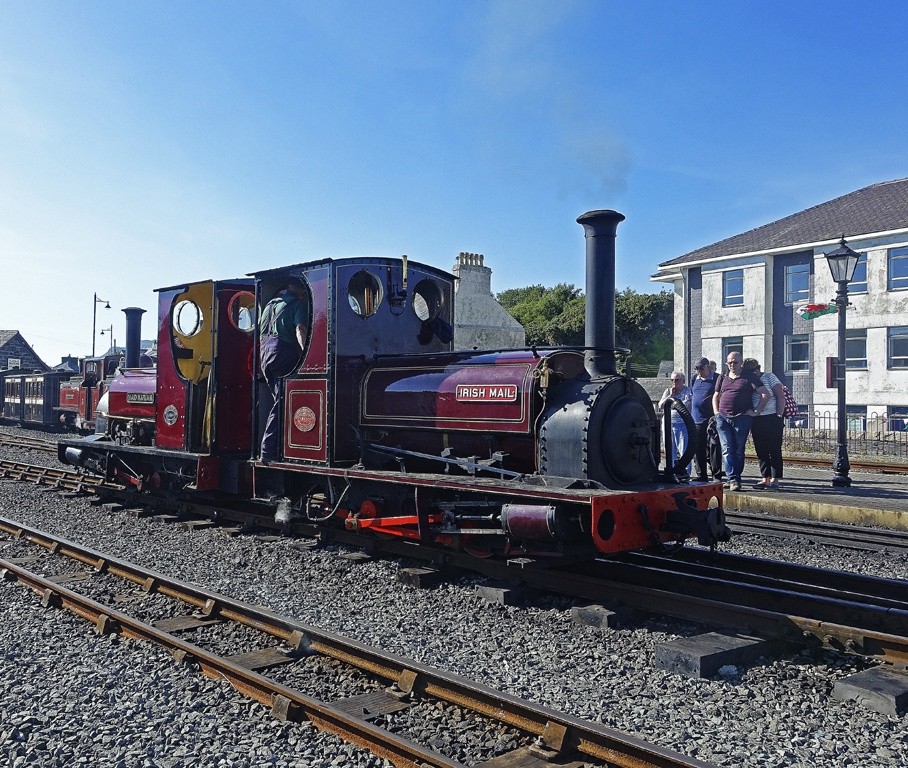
[[261, 452, 280, 464]]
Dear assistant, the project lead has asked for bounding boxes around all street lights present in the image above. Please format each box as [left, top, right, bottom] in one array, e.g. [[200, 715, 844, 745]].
[[92, 292, 113, 371], [819, 233, 864, 489], [99, 324, 113, 355]]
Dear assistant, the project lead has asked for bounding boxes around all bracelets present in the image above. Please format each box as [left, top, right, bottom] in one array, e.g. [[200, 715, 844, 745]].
[[752, 409, 758, 413], [777, 414, 782, 418]]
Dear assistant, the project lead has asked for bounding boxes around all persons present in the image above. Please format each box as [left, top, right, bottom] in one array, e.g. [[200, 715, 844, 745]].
[[711, 351, 770, 491], [658, 370, 693, 477], [692, 356, 722, 483], [742, 357, 786, 490], [260, 278, 311, 463]]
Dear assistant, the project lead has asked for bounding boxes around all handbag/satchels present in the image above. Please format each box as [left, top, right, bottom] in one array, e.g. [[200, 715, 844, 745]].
[[782, 385, 799, 418]]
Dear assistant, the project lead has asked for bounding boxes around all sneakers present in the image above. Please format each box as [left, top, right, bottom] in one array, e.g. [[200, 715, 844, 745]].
[[730, 479, 739, 491], [689, 474, 708, 483], [713, 476, 720, 483], [752, 482, 771, 489], [723, 481, 742, 488], [763, 482, 779, 491]]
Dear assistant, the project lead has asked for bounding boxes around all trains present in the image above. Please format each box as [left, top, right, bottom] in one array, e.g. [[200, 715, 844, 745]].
[[53, 206, 733, 577], [0, 343, 158, 435]]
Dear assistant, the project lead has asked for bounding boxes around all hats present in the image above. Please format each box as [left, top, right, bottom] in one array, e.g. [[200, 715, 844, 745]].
[[694, 357, 709, 366], [742, 358, 760, 370], [289, 277, 306, 288], [665, 371, 685, 380]]
